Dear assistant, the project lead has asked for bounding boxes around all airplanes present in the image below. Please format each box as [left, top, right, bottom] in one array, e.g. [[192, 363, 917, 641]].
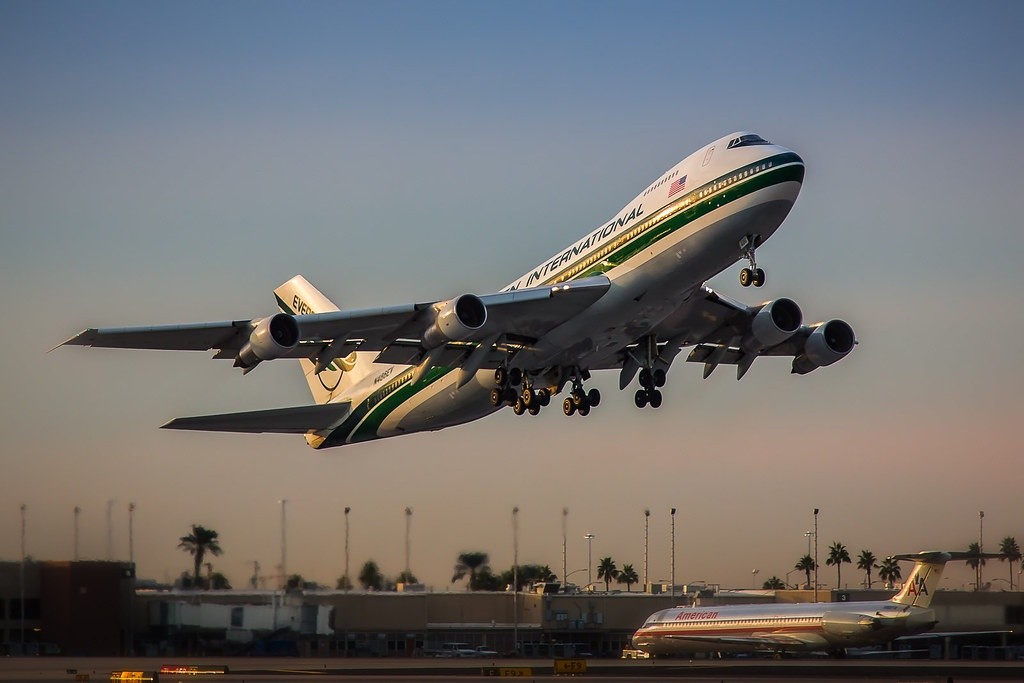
[[631, 549, 1024, 662], [48, 131, 859, 450]]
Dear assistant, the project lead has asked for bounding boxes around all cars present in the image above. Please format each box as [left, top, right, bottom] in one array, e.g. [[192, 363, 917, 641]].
[[450, 643, 497, 658]]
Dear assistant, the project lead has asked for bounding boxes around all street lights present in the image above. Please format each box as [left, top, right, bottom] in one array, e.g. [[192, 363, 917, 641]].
[[813, 509, 819, 603], [512, 508, 519, 650], [404, 507, 412, 584], [584, 533, 596, 583], [803, 530, 813, 556], [561, 508, 568, 587], [978, 511, 984, 591], [644, 510, 650, 584], [670, 508, 676, 596], [343, 506, 351, 580], [18, 497, 137, 658]]
